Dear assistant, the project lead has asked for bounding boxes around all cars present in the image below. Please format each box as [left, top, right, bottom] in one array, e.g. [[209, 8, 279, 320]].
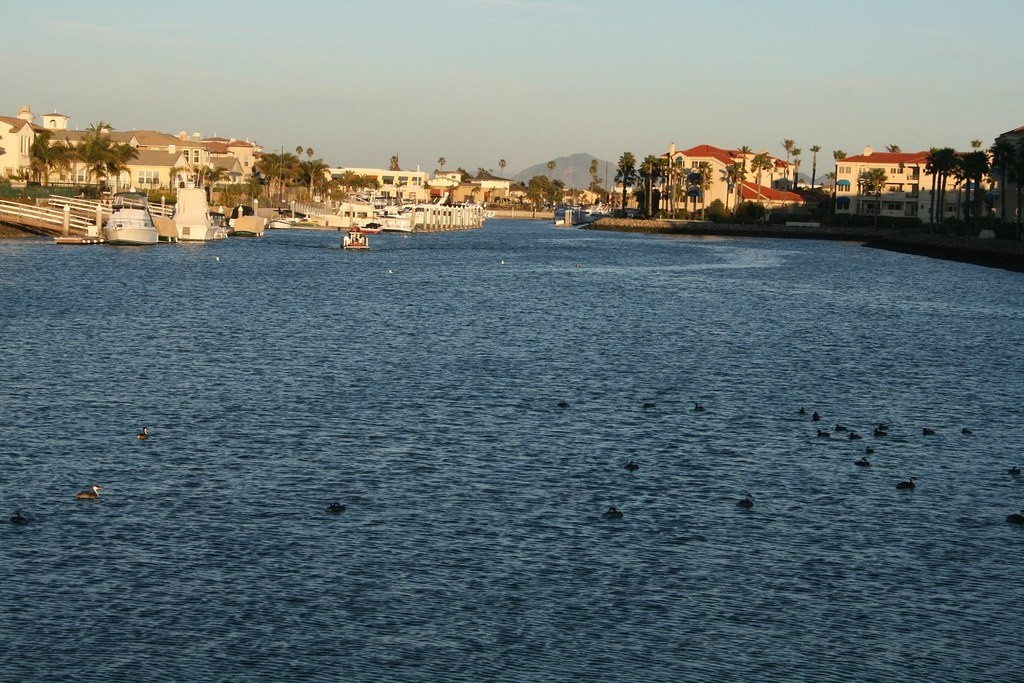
[[625, 208, 641, 219]]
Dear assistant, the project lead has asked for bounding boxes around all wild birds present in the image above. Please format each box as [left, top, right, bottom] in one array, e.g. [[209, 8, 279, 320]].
[[0, 394, 1024, 585]]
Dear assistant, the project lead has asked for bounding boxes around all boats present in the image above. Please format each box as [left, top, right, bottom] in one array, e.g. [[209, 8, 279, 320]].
[[353, 216, 383, 235], [270, 219, 292, 229], [341, 224, 370, 251], [104, 185, 160, 243]]
